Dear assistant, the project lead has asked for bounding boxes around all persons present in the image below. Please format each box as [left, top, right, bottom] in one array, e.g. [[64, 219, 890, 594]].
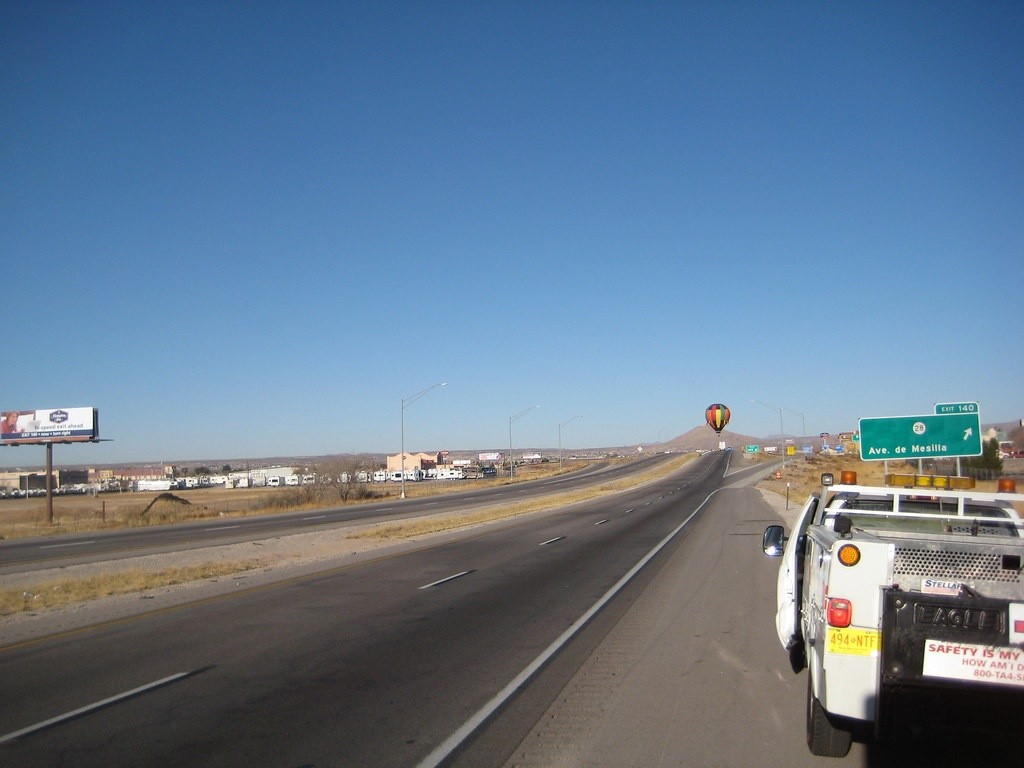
[[1, 409, 25, 434]]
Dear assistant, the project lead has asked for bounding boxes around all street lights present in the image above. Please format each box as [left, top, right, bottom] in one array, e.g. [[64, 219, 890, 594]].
[[559, 415, 583, 468], [750, 400, 806, 469], [400, 382, 448, 498], [509, 405, 541, 478]]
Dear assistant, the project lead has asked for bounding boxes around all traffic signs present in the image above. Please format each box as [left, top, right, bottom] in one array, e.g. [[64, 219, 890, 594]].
[[858, 412, 982, 462]]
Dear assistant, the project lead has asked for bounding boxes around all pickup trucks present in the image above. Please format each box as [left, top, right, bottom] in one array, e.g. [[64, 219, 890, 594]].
[[762, 466, 1022, 757]]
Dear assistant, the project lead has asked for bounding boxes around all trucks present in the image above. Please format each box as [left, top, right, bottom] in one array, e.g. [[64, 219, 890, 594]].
[[719, 441, 726, 451], [138, 468, 466, 491]]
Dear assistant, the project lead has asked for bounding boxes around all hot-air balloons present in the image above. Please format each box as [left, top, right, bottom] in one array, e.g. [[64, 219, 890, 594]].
[[705, 404, 730, 437]]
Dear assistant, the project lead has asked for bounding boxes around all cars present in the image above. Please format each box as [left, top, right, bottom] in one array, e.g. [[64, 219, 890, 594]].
[[727, 447, 732, 450]]
